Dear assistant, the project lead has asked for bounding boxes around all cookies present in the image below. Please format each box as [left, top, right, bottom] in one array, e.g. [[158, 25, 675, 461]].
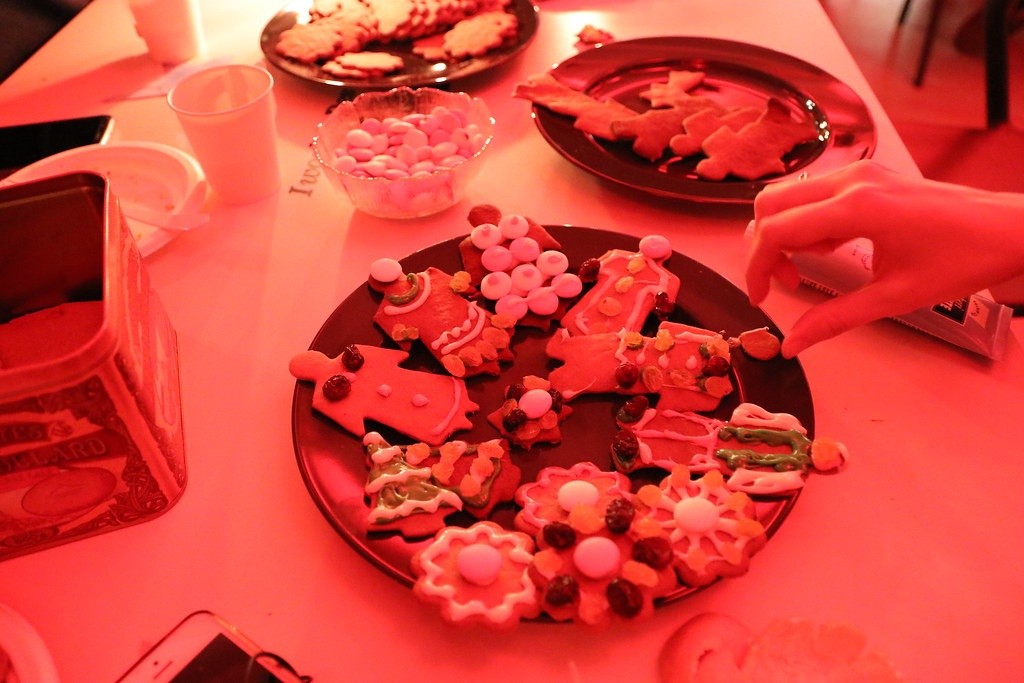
[[291, 206, 848, 627], [512, 62, 822, 177], [275, 1, 517, 84]]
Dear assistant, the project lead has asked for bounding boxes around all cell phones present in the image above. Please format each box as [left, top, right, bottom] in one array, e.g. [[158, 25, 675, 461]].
[[114, 611, 303, 683]]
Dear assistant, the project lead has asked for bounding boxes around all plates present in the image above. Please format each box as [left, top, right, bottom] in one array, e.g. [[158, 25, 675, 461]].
[[259, 3, 537, 88], [530, 33, 877, 214], [291, 226, 815, 620], [0, 141, 208, 262]]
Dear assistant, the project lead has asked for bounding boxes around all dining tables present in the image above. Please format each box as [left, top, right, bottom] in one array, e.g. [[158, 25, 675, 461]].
[[0, 0, 1024, 681]]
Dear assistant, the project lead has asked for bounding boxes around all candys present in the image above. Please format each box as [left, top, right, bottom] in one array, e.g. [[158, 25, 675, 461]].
[[333, 107, 478, 205]]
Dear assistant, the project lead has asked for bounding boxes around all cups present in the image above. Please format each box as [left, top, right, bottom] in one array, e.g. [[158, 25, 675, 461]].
[[167, 65, 284, 205], [125, 0, 211, 65]]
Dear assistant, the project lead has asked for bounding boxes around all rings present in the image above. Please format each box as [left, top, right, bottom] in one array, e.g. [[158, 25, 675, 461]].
[[798, 172, 809, 183]]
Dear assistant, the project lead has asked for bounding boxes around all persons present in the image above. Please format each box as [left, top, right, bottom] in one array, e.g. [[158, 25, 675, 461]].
[[739, 161, 1023, 361]]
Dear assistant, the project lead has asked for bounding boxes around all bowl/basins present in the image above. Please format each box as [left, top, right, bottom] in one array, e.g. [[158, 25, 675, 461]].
[[312, 83, 498, 221]]
